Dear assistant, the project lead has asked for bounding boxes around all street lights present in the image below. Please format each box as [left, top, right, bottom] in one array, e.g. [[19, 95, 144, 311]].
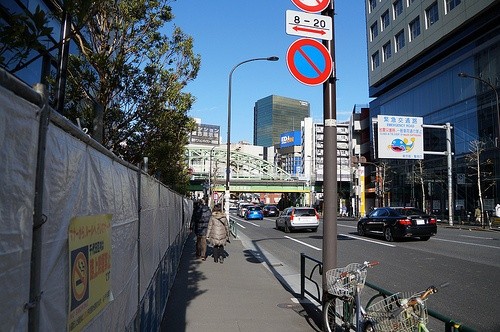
[[225, 56, 280, 221], [208, 143, 232, 196], [458, 72, 500, 207]]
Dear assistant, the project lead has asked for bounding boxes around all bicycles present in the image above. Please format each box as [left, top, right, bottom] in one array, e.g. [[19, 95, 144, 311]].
[[220, 216, 237, 244], [323, 259, 452, 332]]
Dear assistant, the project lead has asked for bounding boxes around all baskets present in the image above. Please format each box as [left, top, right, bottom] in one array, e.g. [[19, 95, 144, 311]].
[[371, 293, 429, 332], [327, 263, 367, 297]]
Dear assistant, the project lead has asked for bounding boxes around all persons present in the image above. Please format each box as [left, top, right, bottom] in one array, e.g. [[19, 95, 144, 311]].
[[495, 200, 500, 218], [190, 199, 212, 260], [207, 205, 229, 262], [342, 204, 347, 216], [475, 207, 481, 225]]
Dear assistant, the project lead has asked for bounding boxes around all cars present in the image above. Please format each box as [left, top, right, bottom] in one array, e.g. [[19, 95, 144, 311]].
[[276, 206, 320, 232], [357, 206, 437, 242], [213, 192, 264, 219], [262, 205, 279, 217], [243, 206, 264, 220]]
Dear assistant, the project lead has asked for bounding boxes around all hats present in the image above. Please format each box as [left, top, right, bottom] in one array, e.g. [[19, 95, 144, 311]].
[[199, 199, 205, 204]]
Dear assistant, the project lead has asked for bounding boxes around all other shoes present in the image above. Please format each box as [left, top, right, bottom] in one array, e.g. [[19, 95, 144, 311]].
[[219, 256, 223, 263], [214, 260, 218, 263], [202, 257, 206, 261]]
